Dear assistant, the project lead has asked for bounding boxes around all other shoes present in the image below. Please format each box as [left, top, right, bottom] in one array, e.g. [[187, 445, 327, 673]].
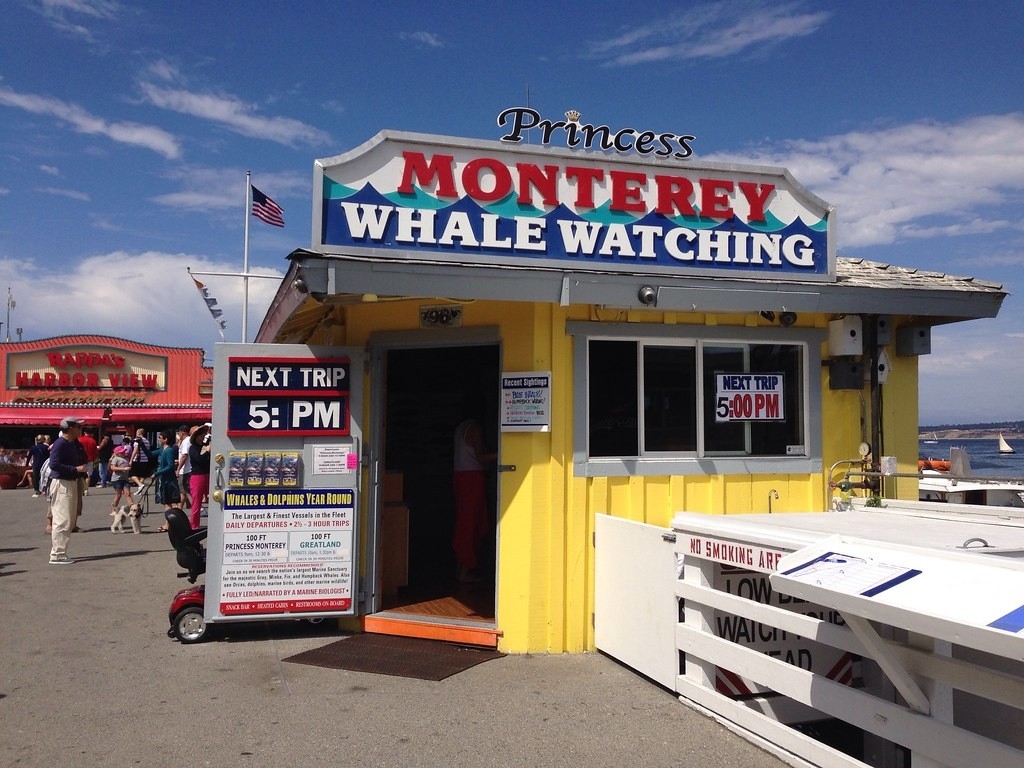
[[32, 493, 39, 497], [157, 527, 168, 533], [136, 484, 145, 495]]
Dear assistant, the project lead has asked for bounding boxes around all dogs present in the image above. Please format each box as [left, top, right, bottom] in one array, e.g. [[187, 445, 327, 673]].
[[111, 502, 143, 535]]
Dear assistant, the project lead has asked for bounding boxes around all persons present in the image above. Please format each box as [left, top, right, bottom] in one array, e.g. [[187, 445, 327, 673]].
[[452, 401, 498, 583], [0, 416, 212, 565]]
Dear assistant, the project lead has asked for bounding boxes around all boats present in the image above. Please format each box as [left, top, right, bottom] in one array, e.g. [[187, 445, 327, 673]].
[[918, 456, 951, 471]]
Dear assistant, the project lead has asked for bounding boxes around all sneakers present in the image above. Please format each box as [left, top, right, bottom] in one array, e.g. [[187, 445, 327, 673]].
[[49, 556, 75, 564]]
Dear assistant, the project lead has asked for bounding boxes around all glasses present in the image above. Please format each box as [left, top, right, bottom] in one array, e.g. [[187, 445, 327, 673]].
[[72, 425, 81, 429], [159, 436, 163, 439], [1, 451, 5, 452]]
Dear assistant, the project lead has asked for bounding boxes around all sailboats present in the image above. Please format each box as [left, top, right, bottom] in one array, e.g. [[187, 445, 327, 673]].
[[999, 432, 1016, 454], [924, 431, 940, 444]]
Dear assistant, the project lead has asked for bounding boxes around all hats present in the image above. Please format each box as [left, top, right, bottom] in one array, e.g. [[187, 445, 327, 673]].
[[114, 446, 127, 453], [176, 425, 190, 433], [190, 425, 209, 444], [60, 416, 85, 428]]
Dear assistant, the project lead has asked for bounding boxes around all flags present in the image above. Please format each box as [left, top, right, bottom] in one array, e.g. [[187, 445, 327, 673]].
[[251, 184, 285, 228]]
